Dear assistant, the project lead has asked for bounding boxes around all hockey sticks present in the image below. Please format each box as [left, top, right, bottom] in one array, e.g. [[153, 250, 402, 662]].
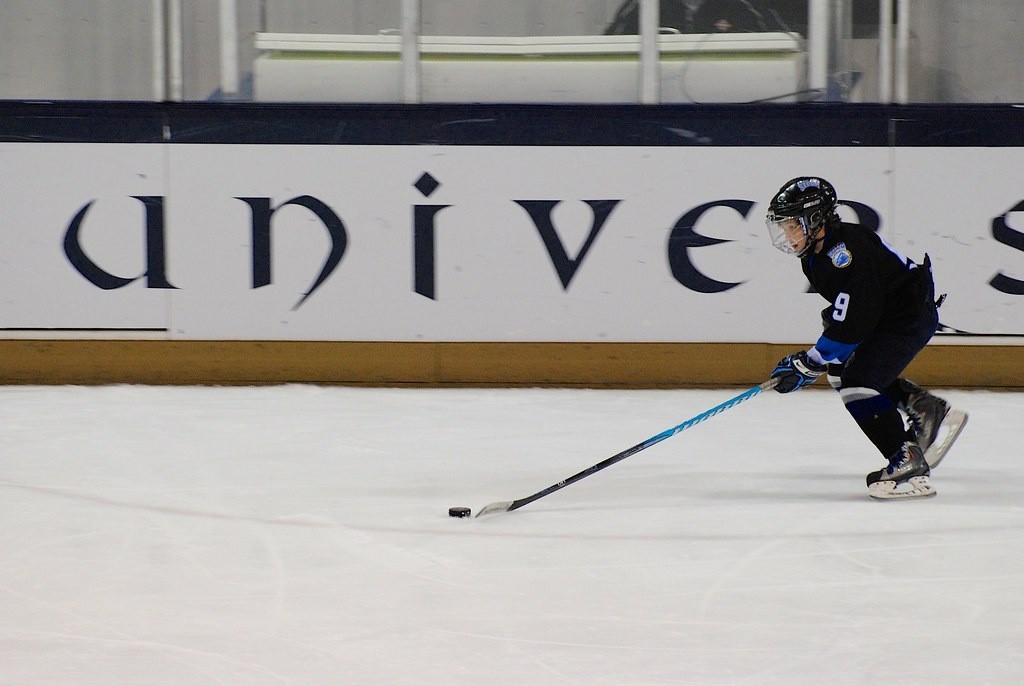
[[477, 370, 784, 522]]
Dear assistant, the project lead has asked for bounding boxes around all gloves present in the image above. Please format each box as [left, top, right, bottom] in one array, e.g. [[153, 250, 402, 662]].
[[770, 349, 828, 394]]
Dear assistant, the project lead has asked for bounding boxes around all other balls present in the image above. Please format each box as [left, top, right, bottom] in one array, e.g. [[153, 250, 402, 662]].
[[449, 507, 472, 519]]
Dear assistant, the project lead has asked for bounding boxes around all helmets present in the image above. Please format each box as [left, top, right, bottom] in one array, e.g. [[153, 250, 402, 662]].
[[765, 176, 838, 230]]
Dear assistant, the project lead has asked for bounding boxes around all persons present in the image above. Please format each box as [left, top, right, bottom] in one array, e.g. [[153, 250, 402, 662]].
[[603, 1, 789, 35], [766, 176, 969, 501]]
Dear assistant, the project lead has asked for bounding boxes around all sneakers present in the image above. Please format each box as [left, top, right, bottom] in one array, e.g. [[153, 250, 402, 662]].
[[866, 441, 937, 502], [904, 389, 969, 471]]
